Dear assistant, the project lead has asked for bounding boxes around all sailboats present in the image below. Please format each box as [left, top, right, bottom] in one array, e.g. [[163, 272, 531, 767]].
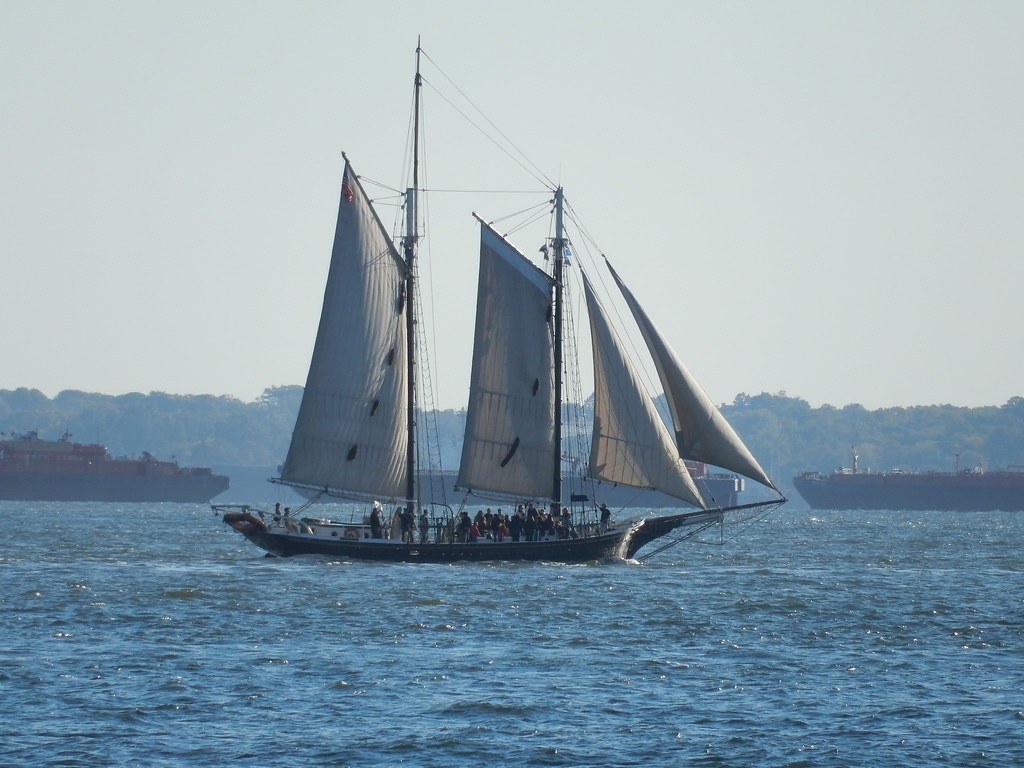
[[210, 28, 788, 564]]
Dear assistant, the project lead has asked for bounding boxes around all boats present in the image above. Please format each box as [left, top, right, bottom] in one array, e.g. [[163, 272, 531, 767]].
[[790, 444, 1023, 514], [4, 425, 233, 504]]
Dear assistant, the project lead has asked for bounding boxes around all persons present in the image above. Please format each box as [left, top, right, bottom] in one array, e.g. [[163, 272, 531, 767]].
[[593, 502, 611, 535], [275, 502, 293, 530], [369, 500, 571, 541]]
